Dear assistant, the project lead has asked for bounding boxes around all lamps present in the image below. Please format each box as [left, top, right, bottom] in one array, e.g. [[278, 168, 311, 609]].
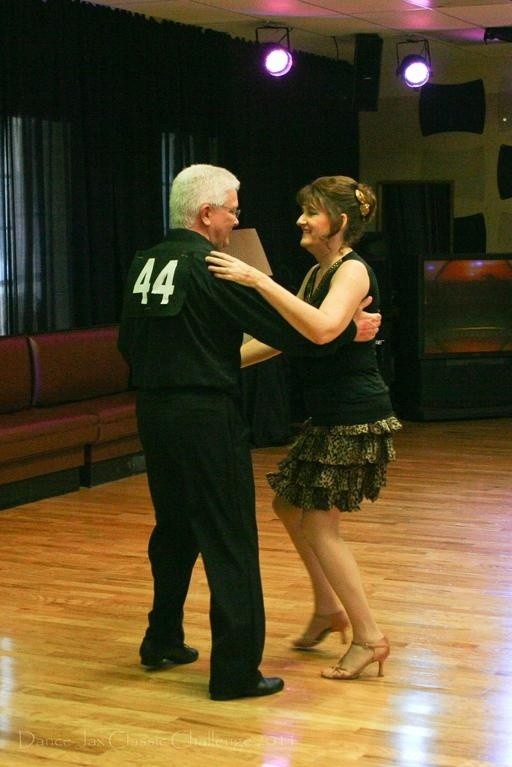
[[395, 38, 434, 92]]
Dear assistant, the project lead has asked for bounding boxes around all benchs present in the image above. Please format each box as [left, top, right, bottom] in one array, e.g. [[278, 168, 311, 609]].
[[0, 324, 146, 510], [254, 25, 294, 77]]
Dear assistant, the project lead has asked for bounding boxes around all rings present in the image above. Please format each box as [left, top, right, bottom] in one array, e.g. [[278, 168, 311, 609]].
[[226, 268, 230, 275]]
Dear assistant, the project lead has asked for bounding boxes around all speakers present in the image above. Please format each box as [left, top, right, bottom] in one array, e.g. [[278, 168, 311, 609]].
[[349, 32, 383, 111]]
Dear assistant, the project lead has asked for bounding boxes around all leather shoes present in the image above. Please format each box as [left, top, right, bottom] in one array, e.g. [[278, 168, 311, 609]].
[[206, 668, 285, 700], [135, 639, 201, 667]]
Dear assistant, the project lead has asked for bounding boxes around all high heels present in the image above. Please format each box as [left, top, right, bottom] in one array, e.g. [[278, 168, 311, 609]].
[[320, 632, 391, 680], [290, 609, 350, 648]]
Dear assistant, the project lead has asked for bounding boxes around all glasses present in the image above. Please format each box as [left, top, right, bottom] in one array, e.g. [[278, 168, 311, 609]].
[[216, 203, 242, 216]]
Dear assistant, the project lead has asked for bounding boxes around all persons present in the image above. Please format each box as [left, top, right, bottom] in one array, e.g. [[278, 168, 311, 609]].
[[117, 163, 385, 701], [204, 175, 405, 682]]
